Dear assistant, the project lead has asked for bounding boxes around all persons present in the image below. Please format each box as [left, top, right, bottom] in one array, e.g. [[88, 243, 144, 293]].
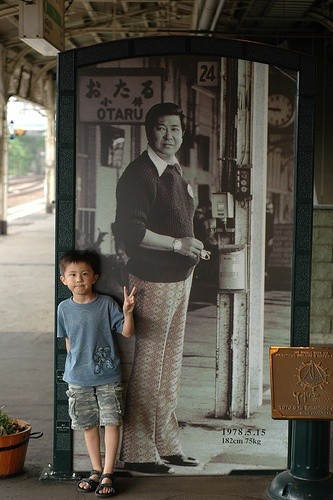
[[111, 102, 204, 473], [57, 250, 136, 497]]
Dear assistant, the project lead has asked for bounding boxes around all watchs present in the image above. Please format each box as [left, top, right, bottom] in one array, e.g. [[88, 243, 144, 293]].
[[173, 237, 183, 252]]
